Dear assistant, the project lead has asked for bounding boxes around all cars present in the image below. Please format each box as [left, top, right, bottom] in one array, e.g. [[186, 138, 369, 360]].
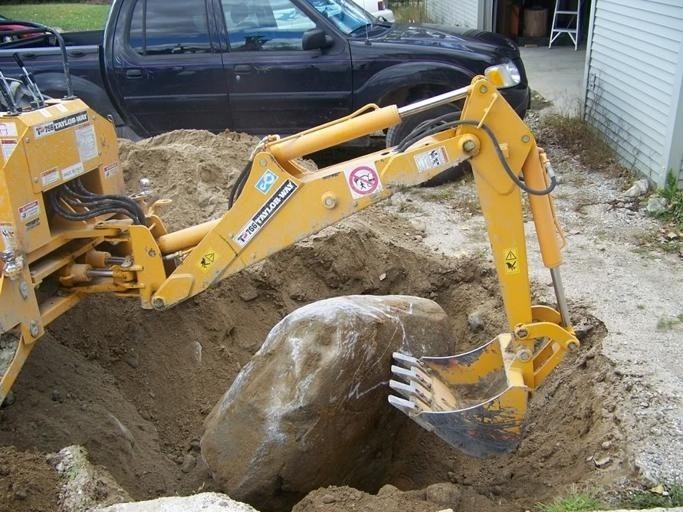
[[215, 1, 399, 31], [1, 12, 61, 44]]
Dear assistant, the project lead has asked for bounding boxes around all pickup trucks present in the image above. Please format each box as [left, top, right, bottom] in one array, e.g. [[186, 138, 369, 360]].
[[0, 1, 533, 187]]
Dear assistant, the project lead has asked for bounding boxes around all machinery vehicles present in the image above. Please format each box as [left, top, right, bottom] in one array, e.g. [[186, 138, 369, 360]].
[[0, 34, 584, 460]]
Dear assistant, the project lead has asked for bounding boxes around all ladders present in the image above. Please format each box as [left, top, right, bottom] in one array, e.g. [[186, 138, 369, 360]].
[[548, 0, 580, 51]]
